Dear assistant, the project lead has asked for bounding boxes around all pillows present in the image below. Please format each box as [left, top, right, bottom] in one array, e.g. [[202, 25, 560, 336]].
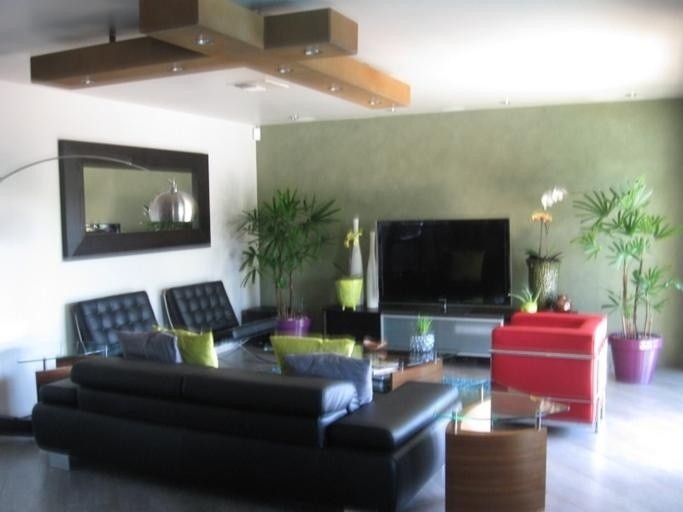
[[265, 334, 356, 373], [279, 352, 374, 409], [149, 322, 218, 371], [113, 326, 181, 363]]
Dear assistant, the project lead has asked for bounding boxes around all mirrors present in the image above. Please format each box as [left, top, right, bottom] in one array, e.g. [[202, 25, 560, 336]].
[[57, 136, 212, 259]]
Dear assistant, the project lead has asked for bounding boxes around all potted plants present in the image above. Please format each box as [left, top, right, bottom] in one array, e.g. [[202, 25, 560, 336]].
[[504, 283, 544, 314], [224, 185, 347, 337], [407, 314, 435, 352], [319, 259, 365, 312], [566, 171, 682, 386]]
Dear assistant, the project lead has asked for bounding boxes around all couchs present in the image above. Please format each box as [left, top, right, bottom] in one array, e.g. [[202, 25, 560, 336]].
[[486, 310, 611, 434], [27, 356, 456, 511]]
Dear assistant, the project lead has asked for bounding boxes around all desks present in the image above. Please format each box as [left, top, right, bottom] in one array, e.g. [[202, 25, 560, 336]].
[[428, 381, 572, 511], [18, 340, 109, 406]]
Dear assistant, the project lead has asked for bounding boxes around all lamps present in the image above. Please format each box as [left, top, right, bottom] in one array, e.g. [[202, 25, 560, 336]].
[[1, 153, 200, 225]]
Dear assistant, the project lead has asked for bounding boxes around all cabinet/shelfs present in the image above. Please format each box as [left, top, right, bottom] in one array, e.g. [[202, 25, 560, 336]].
[[321, 305, 378, 347], [379, 311, 507, 361], [242, 306, 290, 347]]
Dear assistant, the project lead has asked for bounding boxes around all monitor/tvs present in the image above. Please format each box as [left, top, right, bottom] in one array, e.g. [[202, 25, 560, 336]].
[[377, 218, 511, 314]]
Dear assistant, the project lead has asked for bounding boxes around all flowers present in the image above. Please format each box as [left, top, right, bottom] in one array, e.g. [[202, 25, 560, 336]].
[[524, 183, 570, 261]]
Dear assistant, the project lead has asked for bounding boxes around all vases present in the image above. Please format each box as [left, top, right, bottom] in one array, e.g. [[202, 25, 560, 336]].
[[527, 260, 561, 307]]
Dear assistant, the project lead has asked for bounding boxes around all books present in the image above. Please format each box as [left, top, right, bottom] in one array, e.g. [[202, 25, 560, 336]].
[[371, 357, 397, 375]]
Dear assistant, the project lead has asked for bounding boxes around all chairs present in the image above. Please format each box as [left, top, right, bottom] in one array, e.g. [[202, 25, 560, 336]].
[[163, 280, 282, 345], [70, 292, 160, 357]]
